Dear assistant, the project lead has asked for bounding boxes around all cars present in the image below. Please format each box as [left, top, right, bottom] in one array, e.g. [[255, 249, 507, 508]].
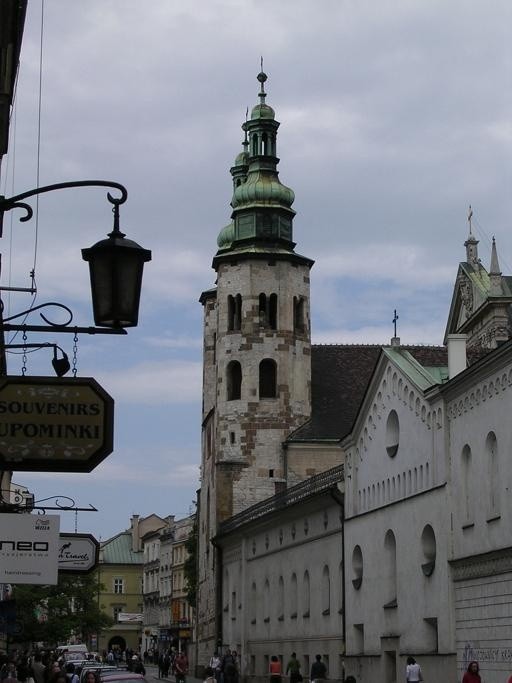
[[56, 644, 148, 683]]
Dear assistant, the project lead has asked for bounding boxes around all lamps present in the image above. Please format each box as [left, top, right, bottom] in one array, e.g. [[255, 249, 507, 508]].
[[1, 176, 154, 337]]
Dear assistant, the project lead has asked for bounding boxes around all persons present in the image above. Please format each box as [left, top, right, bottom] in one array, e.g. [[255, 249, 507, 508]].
[[311, 655, 327, 683], [269, 656, 281, 683], [286, 652, 301, 683], [0, 646, 188, 683], [210, 650, 239, 683], [462, 661, 481, 683], [406, 657, 421, 683]]
